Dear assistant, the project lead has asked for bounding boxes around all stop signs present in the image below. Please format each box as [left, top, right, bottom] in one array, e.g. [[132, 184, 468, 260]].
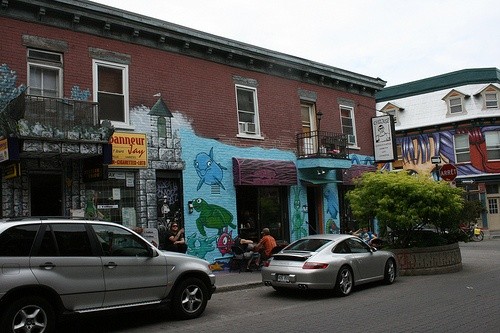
[[440, 163, 457, 181]]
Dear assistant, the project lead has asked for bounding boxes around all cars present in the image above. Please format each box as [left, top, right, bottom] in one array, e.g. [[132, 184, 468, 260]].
[[412, 224, 449, 235]]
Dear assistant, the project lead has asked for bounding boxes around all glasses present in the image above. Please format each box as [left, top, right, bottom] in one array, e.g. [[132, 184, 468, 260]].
[[173, 225, 178, 227]]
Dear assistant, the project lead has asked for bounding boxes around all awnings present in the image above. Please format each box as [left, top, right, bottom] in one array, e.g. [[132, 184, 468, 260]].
[[342, 164, 375, 185], [232, 157, 298, 186]]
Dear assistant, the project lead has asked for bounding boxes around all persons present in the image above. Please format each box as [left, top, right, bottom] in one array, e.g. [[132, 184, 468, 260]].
[[255, 228, 277, 270], [353, 228, 377, 245], [166, 222, 184, 252], [233, 232, 254, 271]]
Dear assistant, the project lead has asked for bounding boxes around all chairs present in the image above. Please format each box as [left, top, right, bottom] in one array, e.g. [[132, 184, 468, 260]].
[[230, 247, 259, 272]]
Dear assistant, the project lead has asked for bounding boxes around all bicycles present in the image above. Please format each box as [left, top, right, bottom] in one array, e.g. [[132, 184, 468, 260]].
[[458, 223, 485, 242]]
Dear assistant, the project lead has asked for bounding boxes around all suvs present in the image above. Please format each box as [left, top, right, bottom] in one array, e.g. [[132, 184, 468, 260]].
[[0, 214, 217, 333]]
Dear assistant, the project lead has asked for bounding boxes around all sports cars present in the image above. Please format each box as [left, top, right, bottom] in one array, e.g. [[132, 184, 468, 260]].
[[260, 233, 399, 297]]
